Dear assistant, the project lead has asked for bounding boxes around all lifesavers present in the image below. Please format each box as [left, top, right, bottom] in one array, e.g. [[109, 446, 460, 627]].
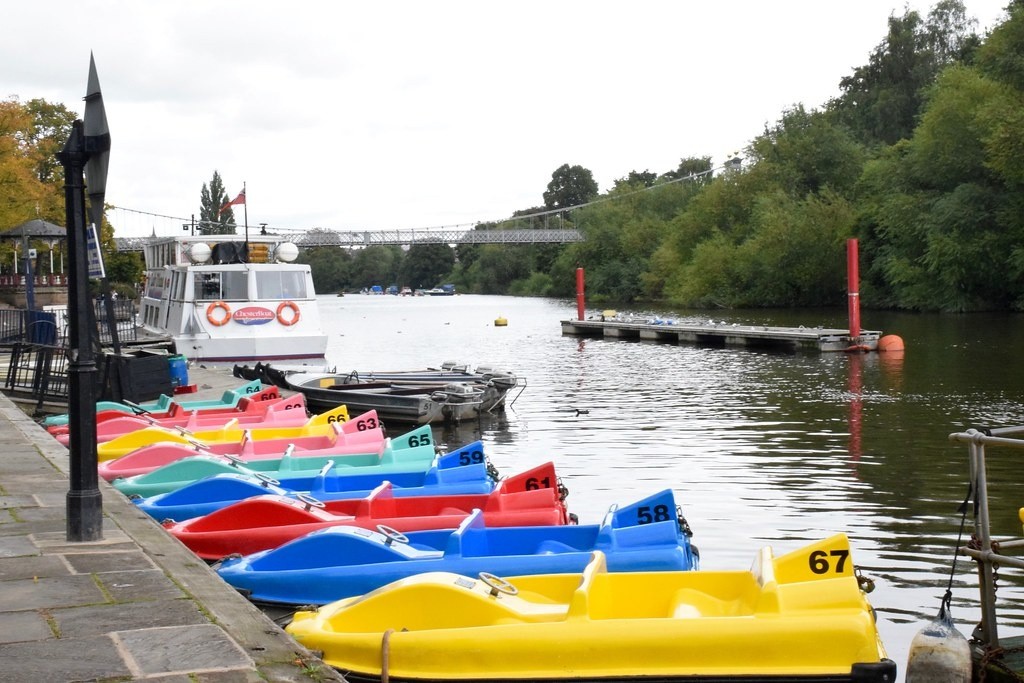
[[206, 302, 231, 326], [277, 302, 300, 326]]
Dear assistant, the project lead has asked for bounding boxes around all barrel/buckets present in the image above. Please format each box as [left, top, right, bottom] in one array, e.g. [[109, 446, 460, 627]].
[[166, 353, 188, 387]]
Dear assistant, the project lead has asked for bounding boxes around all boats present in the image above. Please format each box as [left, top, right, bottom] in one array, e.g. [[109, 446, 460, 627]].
[[209, 487, 704, 610], [131, 214, 330, 364], [160, 461, 575, 567], [37, 377, 500, 524], [233, 360, 529, 429], [284, 524, 896, 683], [359, 283, 457, 297]]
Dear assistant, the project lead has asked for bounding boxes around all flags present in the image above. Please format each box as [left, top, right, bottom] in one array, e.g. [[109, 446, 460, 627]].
[[217, 186, 245, 214]]
